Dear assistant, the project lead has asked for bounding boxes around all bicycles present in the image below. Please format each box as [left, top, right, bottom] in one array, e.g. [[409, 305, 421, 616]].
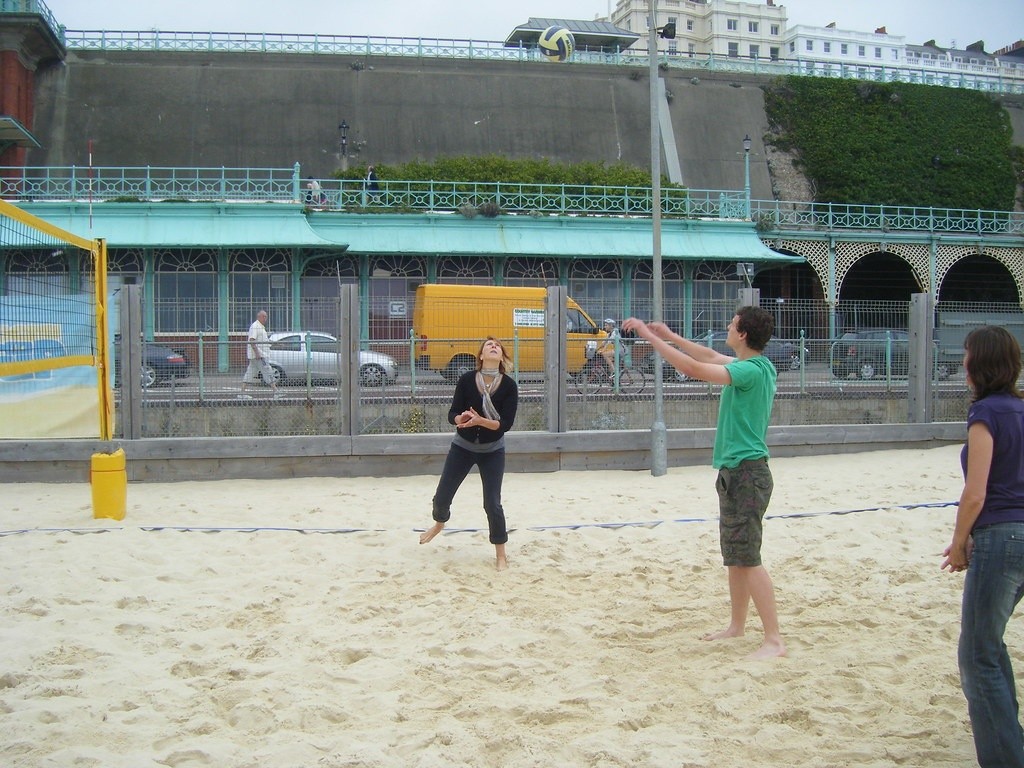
[[575, 345, 645, 394]]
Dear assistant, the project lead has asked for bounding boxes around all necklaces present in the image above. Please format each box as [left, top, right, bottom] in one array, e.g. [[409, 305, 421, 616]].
[[485, 383, 490, 388]]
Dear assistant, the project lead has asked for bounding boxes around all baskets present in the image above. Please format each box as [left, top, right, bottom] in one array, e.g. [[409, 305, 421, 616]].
[[585, 346, 597, 360]]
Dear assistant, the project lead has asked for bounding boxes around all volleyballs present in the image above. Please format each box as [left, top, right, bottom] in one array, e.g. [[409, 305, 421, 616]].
[[537, 25, 576, 63]]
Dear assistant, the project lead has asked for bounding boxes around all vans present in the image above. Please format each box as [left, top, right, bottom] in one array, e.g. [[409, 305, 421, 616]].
[[411, 284, 624, 384]]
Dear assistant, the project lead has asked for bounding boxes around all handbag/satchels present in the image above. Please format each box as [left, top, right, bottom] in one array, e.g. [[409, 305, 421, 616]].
[[586, 341, 597, 359]]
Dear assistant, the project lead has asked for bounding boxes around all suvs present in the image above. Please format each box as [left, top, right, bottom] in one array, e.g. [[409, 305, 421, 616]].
[[827, 329, 959, 379]]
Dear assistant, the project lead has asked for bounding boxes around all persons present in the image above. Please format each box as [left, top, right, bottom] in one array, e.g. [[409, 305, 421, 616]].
[[420, 335, 518, 570], [620, 305, 786, 657], [237, 311, 287, 398], [597, 319, 628, 391], [941, 325, 1024, 768], [306, 176, 327, 205], [366, 166, 379, 204]]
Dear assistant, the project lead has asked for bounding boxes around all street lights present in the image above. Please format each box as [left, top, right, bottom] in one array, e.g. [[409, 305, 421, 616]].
[[339, 119, 349, 172], [743, 134, 752, 221]]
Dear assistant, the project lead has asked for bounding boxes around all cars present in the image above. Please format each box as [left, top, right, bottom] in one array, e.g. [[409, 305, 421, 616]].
[[772, 334, 810, 370], [643, 330, 793, 382], [253, 330, 399, 386], [115, 336, 190, 387]]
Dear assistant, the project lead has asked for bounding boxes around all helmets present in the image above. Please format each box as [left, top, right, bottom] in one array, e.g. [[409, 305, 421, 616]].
[[604, 318, 616, 324]]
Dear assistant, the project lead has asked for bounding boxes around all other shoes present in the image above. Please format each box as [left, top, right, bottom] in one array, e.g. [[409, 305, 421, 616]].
[[609, 372, 616, 378], [273, 394, 287, 399], [237, 394, 252, 399]]
